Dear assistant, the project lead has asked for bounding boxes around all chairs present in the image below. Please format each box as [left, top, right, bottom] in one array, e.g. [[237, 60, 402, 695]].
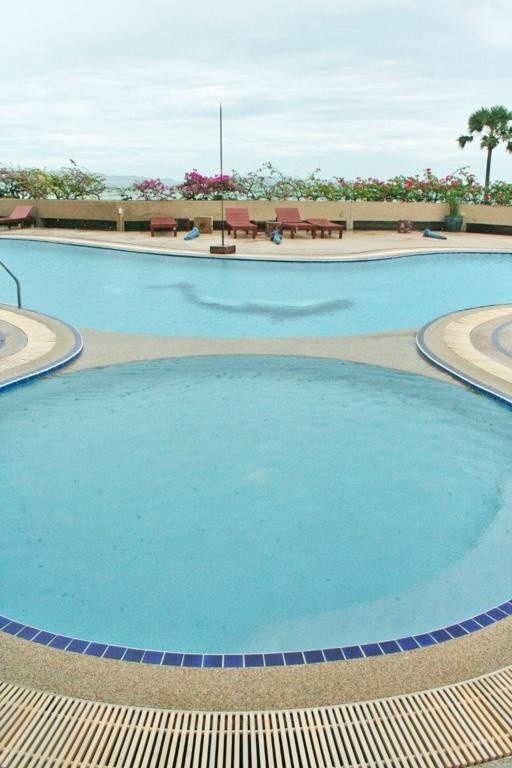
[[225, 207, 344, 239], [0, 205, 33, 228], [151, 217, 177, 237]]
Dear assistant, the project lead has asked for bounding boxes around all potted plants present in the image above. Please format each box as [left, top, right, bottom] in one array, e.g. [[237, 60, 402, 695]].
[[444, 200, 464, 232]]
